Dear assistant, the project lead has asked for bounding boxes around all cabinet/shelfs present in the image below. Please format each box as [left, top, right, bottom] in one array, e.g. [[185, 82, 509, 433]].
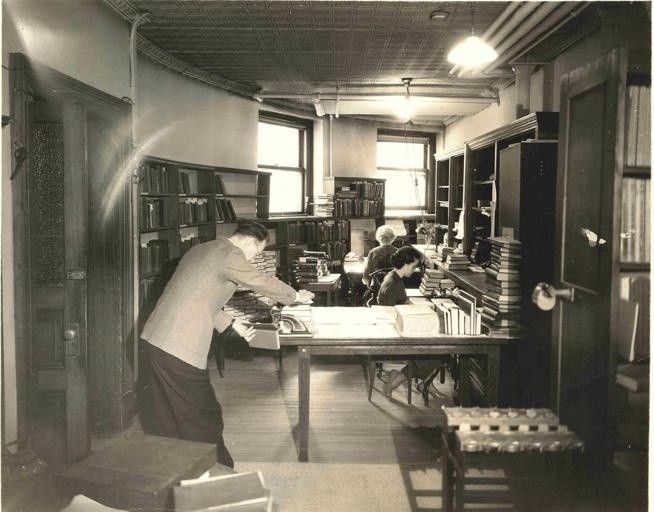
[[605, 22, 648, 369], [269, 215, 433, 304], [137, 159, 272, 305], [433, 108, 556, 266], [321, 176, 386, 216]]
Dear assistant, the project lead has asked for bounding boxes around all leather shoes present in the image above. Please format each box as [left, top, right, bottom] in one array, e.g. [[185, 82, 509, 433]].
[[384, 369, 400, 398], [418, 382, 448, 400]]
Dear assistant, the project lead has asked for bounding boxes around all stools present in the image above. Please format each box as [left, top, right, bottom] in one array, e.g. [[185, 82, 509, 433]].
[[440, 427, 552, 512]]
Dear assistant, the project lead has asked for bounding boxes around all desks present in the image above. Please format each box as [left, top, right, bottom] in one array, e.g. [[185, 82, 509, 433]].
[[296, 303, 521, 463]]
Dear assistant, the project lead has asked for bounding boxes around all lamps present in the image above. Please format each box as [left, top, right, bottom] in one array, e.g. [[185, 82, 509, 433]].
[[446, 2, 500, 70]]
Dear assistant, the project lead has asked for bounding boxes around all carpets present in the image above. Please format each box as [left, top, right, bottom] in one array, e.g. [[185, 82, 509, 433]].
[[228, 458, 529, 511]]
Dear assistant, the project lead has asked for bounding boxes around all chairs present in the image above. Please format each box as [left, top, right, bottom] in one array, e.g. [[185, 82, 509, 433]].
[[363, 295, 421, 405]]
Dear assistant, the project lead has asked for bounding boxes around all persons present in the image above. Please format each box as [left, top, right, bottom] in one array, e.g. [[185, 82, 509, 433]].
[[377, 246, 447, 400], [141, 220, 316, 468], [363, 226, 400, 305]]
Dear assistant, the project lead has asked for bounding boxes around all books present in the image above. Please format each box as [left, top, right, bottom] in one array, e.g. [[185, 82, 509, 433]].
[[618, 85, 649, 448], [396, 234, 524, 339], [172, 464, 274, 512]]
